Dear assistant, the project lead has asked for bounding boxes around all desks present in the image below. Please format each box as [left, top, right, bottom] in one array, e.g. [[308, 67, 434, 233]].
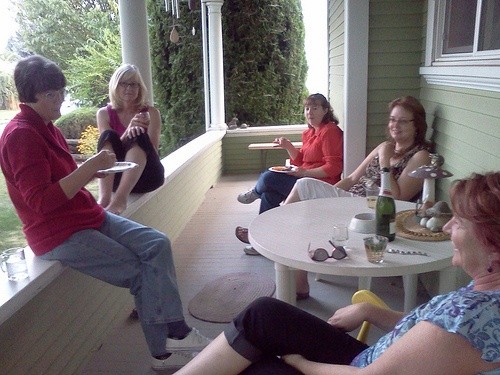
[[248, 142, 302, 173], [248, 197, 460, 318]]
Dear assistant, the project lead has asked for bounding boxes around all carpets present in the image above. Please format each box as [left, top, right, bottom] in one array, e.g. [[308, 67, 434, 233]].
[[189, 271, 275, 323]]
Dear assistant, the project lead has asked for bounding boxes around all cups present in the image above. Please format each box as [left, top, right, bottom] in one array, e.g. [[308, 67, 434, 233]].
[[364, 187, 380, 209], [0, 247, 30, 282], [331, 224, 349, 247], [362, 235, 389, 265]]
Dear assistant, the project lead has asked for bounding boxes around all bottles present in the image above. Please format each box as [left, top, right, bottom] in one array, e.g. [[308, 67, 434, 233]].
[[375, 168, 396, 242]]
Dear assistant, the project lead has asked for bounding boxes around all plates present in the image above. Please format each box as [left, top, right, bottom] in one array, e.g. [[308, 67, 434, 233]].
[[268, 165, 298, 173], [96, 161, 139, 172]]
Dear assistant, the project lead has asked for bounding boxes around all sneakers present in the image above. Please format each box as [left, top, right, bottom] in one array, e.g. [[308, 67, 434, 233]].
[[150, 351, 199, 371], [238, 189, 260, 204], [165, 327, 212, 352], [243, 246, 260, 255]]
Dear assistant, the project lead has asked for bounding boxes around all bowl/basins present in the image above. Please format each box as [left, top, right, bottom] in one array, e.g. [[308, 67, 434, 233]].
[[349, 212, 376, 234]]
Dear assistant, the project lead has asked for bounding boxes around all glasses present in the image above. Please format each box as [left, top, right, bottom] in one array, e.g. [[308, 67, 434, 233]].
[[119, 80, 140, 88], [40, 87, 69, 102], [387, 116, 415, 124], [308, 240, 348, 262]]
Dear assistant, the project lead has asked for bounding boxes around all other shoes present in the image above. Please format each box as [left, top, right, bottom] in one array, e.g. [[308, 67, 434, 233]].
[[235, 226, 250, 244]]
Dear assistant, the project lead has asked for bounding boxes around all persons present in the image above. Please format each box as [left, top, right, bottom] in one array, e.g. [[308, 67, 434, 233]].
[[97, 64, 165, 216], [0, 56, 212, 371], [237, 93, 344, 256], [172, 170, 500, 375], [235, 96, 430, 244]]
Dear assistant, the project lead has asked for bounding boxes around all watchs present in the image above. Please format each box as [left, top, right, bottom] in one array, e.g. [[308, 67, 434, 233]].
[[379, 167, 392, 172]]
